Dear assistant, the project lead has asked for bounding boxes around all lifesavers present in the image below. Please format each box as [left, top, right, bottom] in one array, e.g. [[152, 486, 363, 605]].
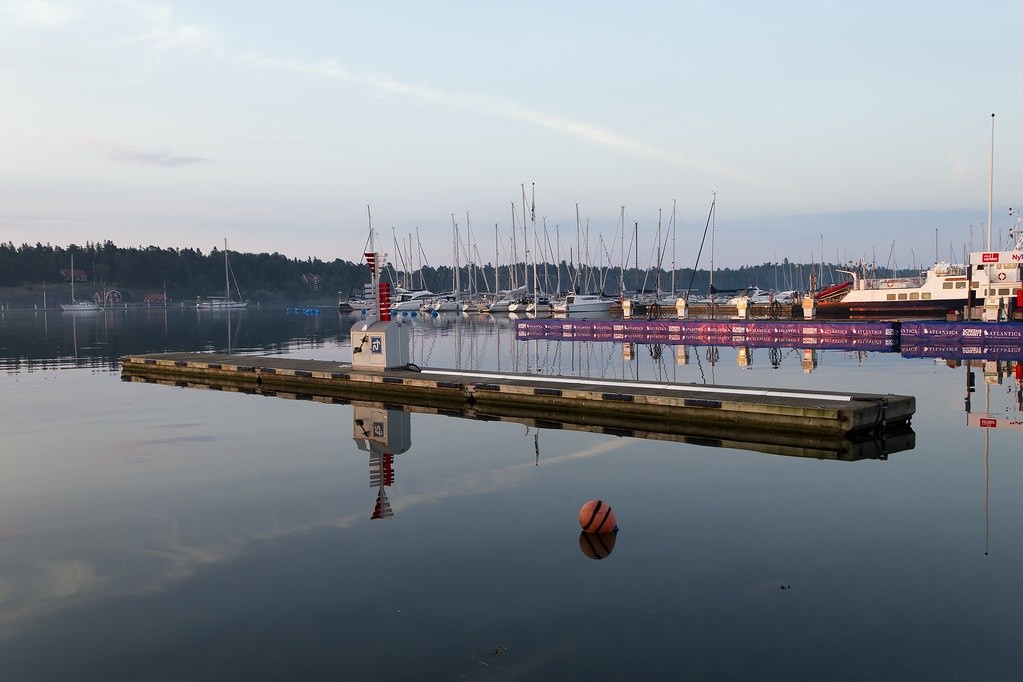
[[998, 273, 1006, 280], [887, 280, 894, 287]]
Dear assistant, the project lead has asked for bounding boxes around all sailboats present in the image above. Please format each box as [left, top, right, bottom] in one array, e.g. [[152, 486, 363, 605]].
[[58, 253, 97, 311], [197, 239, 250, 309], [336, 111, 1022, 324]]
